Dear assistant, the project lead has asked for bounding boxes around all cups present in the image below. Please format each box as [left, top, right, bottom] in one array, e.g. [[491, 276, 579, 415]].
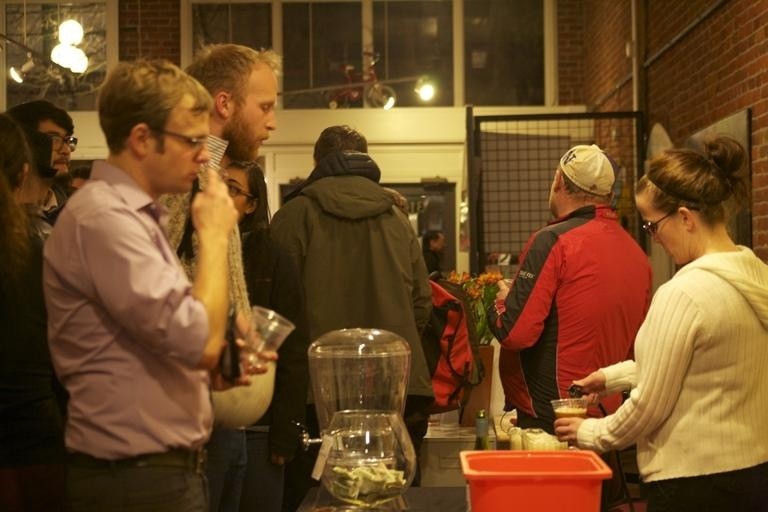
[[549, 399, 588, 445], [242, 305, 296, 368]]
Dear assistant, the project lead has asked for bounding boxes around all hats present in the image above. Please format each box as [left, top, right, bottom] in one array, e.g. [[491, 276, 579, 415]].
[[558, 144, 616, 198]]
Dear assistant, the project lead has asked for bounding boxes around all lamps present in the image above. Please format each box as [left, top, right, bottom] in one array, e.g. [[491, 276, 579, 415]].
[[11, 59, 38, 83]]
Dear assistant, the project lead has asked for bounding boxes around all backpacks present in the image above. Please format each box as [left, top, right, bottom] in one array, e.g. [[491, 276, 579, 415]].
[[421, 277, 483, 417]]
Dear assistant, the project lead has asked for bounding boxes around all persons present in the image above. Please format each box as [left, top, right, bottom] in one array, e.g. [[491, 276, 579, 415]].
[[271, 126, 433, 485], [217, 160, 271, 314], [0, 96, 94, 512], [151, 43, 279, 511], [43, 61, 239, 509], [423, 229, 444, 279], [486, 144, 654, 511], [553, 135, 767, 512]]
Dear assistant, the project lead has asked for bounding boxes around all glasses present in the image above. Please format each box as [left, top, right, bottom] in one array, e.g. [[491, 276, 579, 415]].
[[49, 135, 78, 153], [226, 184, 255, 202], [161, 130, 206, 149], [219, 305, 241, 384], [639, 206, 679, 237]]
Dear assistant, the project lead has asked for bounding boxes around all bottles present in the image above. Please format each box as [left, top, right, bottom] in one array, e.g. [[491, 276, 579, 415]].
[[472, 409, 490, 450]]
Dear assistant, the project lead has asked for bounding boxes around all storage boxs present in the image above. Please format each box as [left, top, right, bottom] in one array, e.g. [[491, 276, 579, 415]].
[[458, 450, 612, 512]]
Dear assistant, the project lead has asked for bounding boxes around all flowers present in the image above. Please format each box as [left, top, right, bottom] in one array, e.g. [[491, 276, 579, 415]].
[[444, 271, 503, 346]]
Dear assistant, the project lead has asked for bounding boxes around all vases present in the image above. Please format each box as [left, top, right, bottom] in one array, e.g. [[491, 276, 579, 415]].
[[459, 344, 492, 424]]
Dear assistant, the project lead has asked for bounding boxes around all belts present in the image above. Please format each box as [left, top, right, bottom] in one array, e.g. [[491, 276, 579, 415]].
[[68, 448, 208, 473]]
